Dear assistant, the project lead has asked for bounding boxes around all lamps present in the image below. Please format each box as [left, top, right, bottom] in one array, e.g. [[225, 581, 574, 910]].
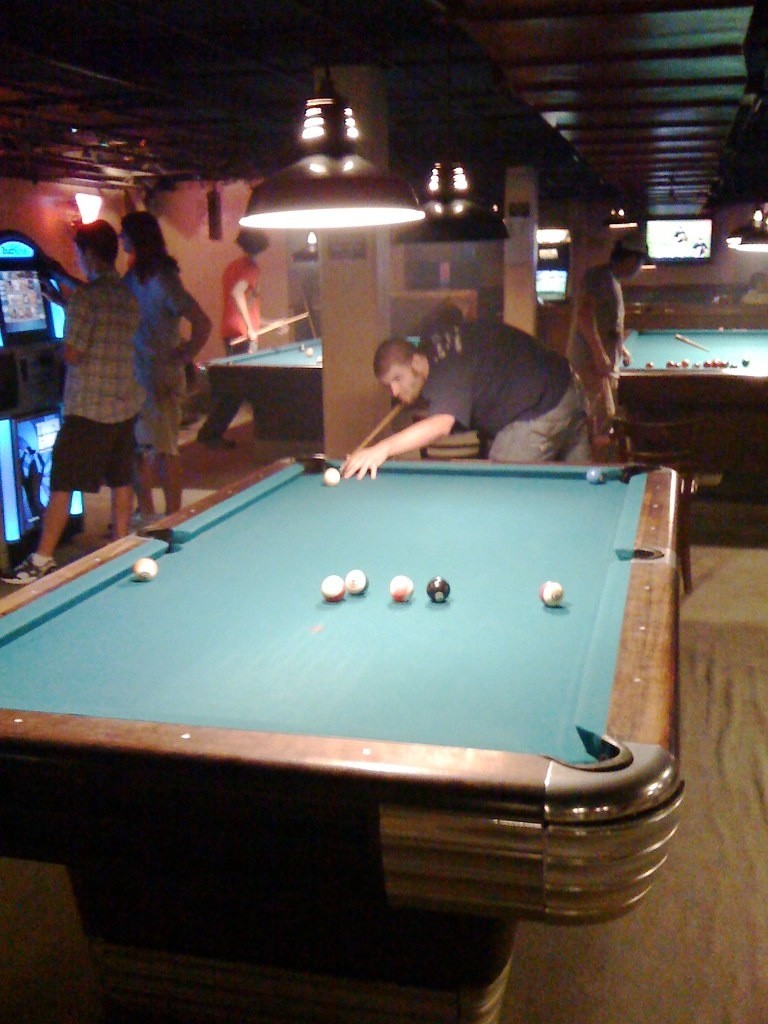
[[725, 201, 768, 252], [239, 91, 648, 232]]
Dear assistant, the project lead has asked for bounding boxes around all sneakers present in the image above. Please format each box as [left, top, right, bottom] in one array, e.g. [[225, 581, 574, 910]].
[[0, 553, 57, 584]]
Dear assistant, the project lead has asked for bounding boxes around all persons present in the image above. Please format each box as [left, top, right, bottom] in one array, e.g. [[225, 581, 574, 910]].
[[337, 304, 585, 481], [197, 227, 291, 450], [0, 209, 215, 586], [674, 226, 709, 256], [564, 237, 646, 466]]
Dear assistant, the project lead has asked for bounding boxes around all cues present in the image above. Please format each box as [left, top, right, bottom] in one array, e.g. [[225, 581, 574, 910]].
[[675, 333, 710, 352], [227, 312, 309, 346], [338, 402, 404, 476]]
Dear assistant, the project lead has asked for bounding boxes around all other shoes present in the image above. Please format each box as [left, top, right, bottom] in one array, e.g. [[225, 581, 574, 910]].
[[202, 437, 237, 451]]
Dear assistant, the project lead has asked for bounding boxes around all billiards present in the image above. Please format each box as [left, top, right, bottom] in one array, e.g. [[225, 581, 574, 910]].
[[586, 468, 605, 485], [321, 575, 346, 602], [623, 357, 750, 368], [345, 569, 369, 595], [427, 575, 450, 603], [389, 575, 414, 603], [299, 344, 322, 364], [323, 468, 340, 487], [132, 557, 159, 580], [539, 580, 564, 607]]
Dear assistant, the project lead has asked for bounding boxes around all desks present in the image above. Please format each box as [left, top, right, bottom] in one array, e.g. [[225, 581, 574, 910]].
[[194, 331, 421, 444], [614, 319, 768, 509], [0, 459, 695, 1024]]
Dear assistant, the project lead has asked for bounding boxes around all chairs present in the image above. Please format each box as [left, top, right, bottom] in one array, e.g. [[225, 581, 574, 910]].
[[609, 415, 700, 594]]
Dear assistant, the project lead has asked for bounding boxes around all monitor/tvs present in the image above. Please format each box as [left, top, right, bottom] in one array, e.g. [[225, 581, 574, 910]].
[[646, 218, 714, 262], [0, 257, 55, 343]]
